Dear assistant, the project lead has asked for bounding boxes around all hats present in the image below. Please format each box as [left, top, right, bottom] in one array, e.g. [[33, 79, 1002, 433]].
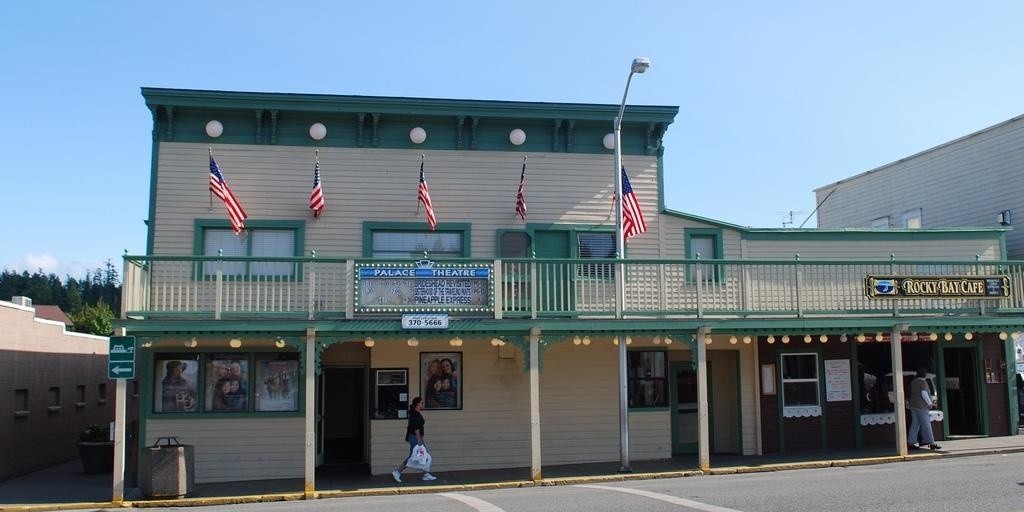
[[165, 361, 188, 375]]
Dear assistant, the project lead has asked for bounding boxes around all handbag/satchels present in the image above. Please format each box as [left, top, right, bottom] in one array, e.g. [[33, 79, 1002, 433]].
[[405, 425, 411, 443]]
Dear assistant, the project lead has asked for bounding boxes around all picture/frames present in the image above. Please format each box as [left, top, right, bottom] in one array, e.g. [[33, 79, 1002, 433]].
[[419, 351, 463, 410], [152, 351, 300, 414]]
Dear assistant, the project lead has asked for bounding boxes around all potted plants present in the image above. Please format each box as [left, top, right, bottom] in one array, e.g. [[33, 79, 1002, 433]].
[[76, 423, 114, 475]]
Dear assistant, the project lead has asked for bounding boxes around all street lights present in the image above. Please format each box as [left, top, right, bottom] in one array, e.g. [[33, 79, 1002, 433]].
[[614, 57, 650, 473]]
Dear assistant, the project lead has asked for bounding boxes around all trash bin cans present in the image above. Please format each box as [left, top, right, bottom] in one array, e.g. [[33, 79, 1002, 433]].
[[141, 437, 195, 500]]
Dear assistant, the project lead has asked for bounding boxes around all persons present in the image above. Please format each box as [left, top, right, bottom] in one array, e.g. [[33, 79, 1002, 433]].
[[424, 375, 443, 408], [162, 360, 196, 412], [206, 358, 247, 412], [440, 358, 458, 381], [436, 373, 457, 407], [424, 358, 442, 391], [907, 370, 942, 452], [391, 396, 437, 483]]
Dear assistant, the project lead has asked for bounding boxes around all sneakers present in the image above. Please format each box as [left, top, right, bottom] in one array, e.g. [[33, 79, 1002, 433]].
[[393, 469, 402, 483], [930, 444, 942, 450], [909, 444, 920, 450], [422, 472, 438, 482]]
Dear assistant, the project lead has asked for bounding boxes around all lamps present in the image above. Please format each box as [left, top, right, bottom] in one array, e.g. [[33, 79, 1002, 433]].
[[603, 133, 615, 150], [410, 127, 427, 144], [142, 332, 1019, 348], [309, 123, 327, 140], [509, 129, 526, 145], [205, 120, 224, 138]]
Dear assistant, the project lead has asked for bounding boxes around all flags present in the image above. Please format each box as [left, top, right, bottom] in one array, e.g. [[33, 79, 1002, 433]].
[[208, 153, 248, 235], [515, 162, 528, 221], [417, 162, 437, 232], [612, 164, 647, 240], [308, 160, 325, 217]]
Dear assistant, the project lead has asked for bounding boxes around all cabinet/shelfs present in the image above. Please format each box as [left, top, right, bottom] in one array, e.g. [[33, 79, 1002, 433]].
[[984, 353, 1008, 383]]
